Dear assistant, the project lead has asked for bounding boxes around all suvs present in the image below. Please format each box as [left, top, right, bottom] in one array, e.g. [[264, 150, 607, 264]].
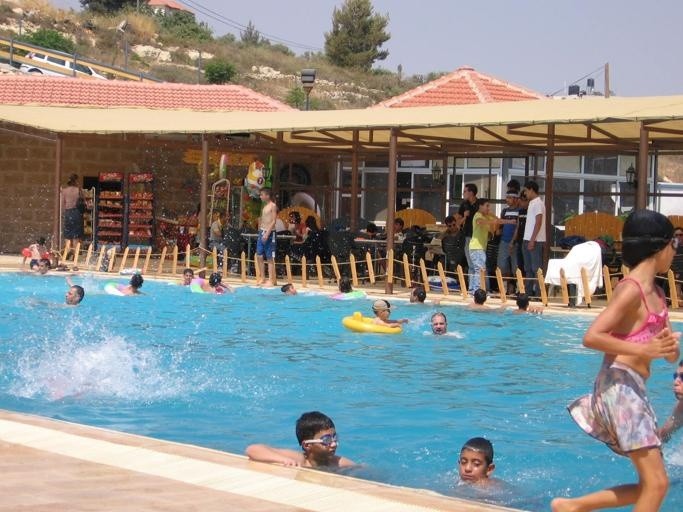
[[17, 48, 109, 92]]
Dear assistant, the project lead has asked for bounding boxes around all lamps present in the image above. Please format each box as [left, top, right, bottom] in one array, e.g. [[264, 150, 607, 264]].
[[626, 162, 638, 191], [432, 162, 445, 186]]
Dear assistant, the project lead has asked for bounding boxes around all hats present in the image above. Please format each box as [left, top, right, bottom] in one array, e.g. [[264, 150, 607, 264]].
[[372, 300, 397, 310]]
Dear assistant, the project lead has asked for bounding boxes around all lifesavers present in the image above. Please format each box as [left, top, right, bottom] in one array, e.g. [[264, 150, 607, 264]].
[[190, 278, 235, 293], [105, 283, 134, 296], [196, 156, 219, 182], [120, 268, 139, 276], [291, 192, 320, 216], [331, 291, 366, 299], [342, 312, 401, 335], [218, 154, 228, 178]]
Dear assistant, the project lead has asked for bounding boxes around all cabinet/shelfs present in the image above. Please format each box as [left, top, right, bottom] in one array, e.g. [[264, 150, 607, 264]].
[[95, 172, 125, 256], [125, 173, 153, 258], [59, 184, 94, 254]]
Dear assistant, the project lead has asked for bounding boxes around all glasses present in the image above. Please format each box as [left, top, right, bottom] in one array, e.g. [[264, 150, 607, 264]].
[[621, 238, 679, 250], [305, 434, 337, 445], [673, 372, 682, 380], [448, 224, 456, 227]]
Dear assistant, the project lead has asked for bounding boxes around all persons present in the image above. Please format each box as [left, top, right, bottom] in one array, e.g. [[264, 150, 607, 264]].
[[200, 272, 236, 295], [469, 197, 519, 299], [657, 359, 683, 444], [430, 312, 450, 336], [496, 190, 527, 297], [671, 227, 683, 298], [409, 287, 440, 305], [512, 293, 545, 315], [371, 300, 408, 327], [443, 216, 460, 235], [464, 288, 507, 313], [245, 411, 389, 484], [20, 238, 55, 273], [377, 218, 404, 276], [516, 191, 530, 285], [457, 183, 492, 293], [249, 187, 277, 288], [361, 223, 378, 276], [456, 436, 546, 511], [60, 174, 84, 262], [550, 208, 683, 511], [287, 189, 321, 218], [175, 269, 195, 286], [285, 216, 322, 276], [31, 277, 83, 308], [523, 181, 546, 301], [120, 272, 151, 296], [280, 283, 297, 295], [210, 211, 235, 271], [32, 258, 55, 277], [506, 179, 520, 195], [287, 212, 305, 242], [337, 277, 352, 293]]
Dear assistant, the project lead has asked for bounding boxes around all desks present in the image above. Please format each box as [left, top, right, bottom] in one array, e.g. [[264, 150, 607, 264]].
[[354, 240, 403, 278], [549, 247, 621, 306], [240, 233, 296, 277]]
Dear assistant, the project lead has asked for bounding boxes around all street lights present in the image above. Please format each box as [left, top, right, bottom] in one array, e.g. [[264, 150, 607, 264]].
[[300, 68, 316, 110]]
